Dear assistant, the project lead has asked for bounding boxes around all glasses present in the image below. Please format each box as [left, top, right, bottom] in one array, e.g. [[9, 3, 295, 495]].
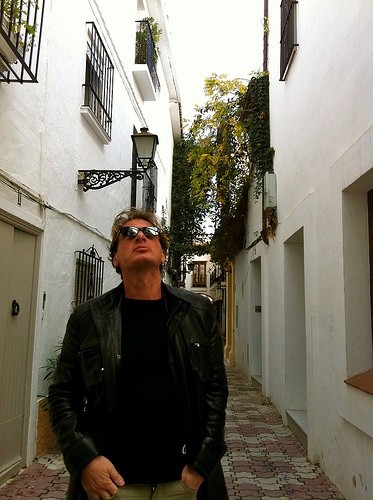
[[115, 226, 162, 239]]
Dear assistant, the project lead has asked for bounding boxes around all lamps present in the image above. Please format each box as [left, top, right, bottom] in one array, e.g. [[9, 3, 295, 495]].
[[78, 127, 159, 192]]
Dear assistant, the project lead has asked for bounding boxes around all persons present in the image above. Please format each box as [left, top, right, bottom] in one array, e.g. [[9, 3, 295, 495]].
[[47, 206, 230, 500]]
